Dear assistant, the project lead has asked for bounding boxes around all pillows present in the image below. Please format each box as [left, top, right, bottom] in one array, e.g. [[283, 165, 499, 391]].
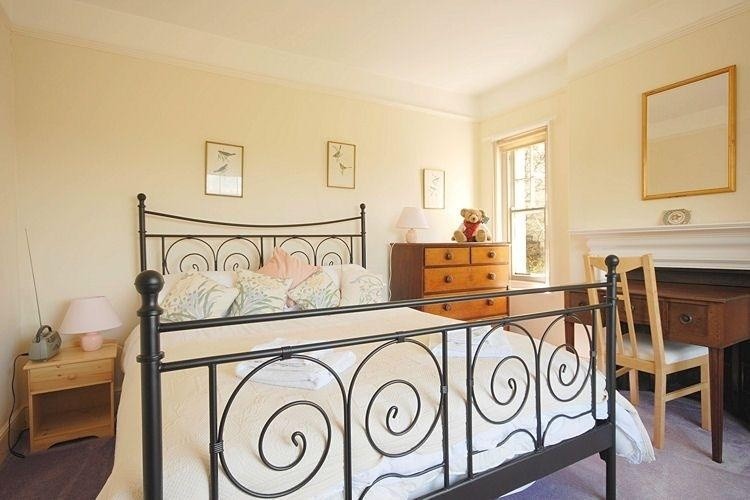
[[157, 244, 388, 324]]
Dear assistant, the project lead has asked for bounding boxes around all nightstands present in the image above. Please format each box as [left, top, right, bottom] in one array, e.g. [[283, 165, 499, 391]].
[[22, 342, 118, 454]]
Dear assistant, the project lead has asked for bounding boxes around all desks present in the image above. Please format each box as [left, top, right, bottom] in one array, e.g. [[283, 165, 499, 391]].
[[562, 278, 750, 463]]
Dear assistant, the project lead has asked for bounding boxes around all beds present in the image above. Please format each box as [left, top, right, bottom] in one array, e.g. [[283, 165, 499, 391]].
[[133, 191, 621, 500]]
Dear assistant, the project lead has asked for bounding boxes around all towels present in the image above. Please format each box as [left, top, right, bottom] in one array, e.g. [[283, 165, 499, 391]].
[[235, 335, 357, 388]]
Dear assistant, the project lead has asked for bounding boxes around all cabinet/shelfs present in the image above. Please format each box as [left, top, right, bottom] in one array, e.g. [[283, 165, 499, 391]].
[[390, 241, 512, 334]]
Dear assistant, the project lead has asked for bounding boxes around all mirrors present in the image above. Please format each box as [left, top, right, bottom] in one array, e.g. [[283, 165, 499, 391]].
[[641, 63, 738, 201]]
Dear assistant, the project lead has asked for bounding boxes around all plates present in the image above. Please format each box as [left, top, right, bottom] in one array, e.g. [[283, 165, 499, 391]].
[[663, 209, 691, 226]]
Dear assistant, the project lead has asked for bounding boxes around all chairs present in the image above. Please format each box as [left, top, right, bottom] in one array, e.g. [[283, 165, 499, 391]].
[[582, 251, 712, 449]]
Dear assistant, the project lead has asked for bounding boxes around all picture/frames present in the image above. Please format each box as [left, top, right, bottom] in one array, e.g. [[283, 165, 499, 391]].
[[423, 166, 447, 210], [206, 140, 245, 198], [326, 140, 357, 190]]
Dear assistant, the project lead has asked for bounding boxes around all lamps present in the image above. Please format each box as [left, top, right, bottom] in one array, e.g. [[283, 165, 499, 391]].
[[397, 205, 428, 243], [60, 296, 122, 351]]
